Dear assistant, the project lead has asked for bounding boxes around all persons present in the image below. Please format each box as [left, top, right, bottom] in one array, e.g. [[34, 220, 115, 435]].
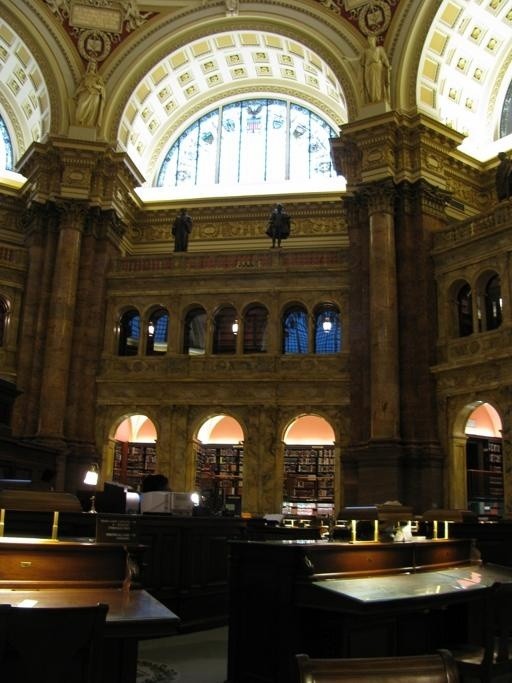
[[143, 474, 172, 492], [494, 152, 512, 202], [265, 203, 291, 248], [171, 208, 192, 251]]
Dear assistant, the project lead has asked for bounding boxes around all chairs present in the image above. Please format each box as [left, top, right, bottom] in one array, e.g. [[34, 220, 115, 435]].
[[1, 601, 110, 683], [451, 582, 511, 683]]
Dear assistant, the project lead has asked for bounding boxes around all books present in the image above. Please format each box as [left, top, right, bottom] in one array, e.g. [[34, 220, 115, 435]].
[[488, 442, 504, 498], [283, 449, 336, 500], [201, 448, 244, 496], [113, 446, 156, 484]]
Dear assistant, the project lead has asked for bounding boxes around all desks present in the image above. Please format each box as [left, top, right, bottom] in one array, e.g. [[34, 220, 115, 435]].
[[307, 557, 512, 657], [1, 579, 181, 683]]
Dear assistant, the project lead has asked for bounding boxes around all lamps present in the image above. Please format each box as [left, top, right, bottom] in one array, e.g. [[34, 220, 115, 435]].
[[83, 464, 100, 513]]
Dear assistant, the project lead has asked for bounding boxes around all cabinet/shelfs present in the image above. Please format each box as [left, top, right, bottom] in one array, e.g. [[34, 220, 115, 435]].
[[464, 434, 503, 517], [111, 434, 335, 504]]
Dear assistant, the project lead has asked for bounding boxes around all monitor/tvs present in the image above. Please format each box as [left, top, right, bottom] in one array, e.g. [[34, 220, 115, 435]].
[[221, 494, 242, 516]]
